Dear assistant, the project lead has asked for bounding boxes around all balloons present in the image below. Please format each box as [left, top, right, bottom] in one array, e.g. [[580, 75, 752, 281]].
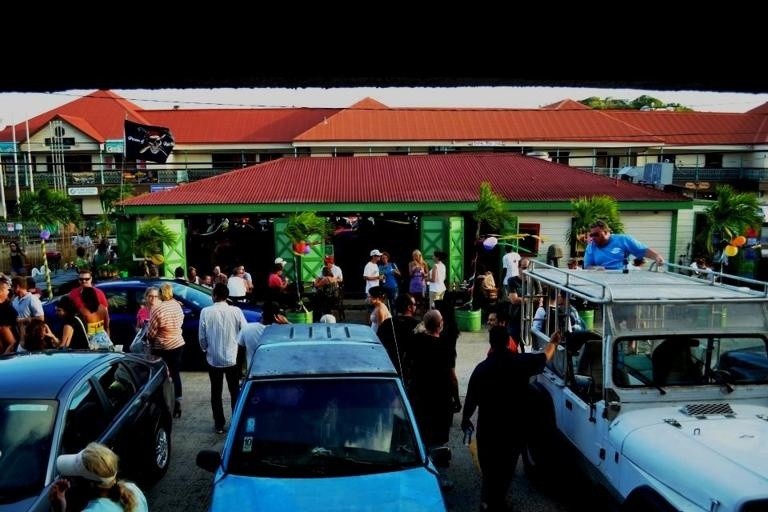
[[724, 227, 758, 259]]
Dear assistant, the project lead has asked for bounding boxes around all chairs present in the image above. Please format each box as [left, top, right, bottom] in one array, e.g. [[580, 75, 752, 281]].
[[577, 340, 618, 398]]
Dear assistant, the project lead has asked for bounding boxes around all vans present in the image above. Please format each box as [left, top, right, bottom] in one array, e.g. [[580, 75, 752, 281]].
[[204, 322, 452, 512]]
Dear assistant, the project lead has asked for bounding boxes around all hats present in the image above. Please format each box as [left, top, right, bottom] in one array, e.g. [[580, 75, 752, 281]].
[[567, 258, 578, 266], [370, 249, 383, 257], [518, 257, 530, 268], [275, 257, 287, 265], [57, 448, 117, 482], [262, 303, 284, 313]]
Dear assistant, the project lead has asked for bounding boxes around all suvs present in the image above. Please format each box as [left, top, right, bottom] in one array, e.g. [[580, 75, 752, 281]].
[[515, 256, 768, 512]]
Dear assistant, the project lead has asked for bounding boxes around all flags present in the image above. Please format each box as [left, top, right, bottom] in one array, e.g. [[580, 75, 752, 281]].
[[121, 119, 175, 164]]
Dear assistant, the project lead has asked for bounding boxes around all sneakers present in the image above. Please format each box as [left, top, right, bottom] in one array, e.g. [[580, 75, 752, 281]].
[[212, 425, 224, 435]]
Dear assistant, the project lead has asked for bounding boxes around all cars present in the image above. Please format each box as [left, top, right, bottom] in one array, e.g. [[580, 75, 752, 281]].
[[0, 350, 176, 511], [41, 278, 266, 364]]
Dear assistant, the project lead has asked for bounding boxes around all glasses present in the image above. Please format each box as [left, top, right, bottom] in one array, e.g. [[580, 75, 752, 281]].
[[414, 303, 417, 306], [79, 275, 92, 280], [590, 229, 605, 237]]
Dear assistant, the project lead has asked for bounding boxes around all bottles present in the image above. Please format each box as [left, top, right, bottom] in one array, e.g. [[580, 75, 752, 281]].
[[380, 271, 386, 284], [463, 427, 472, 447], [622, 251, 629, 273]]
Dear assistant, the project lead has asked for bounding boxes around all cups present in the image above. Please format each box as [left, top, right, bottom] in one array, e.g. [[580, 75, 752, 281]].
[[391, 269, 396, 274]]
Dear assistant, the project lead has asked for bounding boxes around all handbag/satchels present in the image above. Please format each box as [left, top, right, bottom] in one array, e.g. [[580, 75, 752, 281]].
[[394, 272, 404, 288], [129, 326, 153, 355]]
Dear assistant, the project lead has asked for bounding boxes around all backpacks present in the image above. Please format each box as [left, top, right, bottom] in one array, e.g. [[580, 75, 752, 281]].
[[409, 267, 427, 293], [541, 305, 566, 337]]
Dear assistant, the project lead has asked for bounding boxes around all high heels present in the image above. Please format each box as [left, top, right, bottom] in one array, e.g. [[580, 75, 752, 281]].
[[174, 401, 182, 417]]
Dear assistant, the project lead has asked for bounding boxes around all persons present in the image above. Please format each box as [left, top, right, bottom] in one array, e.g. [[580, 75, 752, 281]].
[[147, 255, 163, 277], [0, 240, 111, 349], [134, 284, 184, 418], [689, 257, 714, 281], [49, 443, 148, 512], [267, 249, 461, 450], [171, 265, 252, 304], [472, 220, 664, 357], [461, 325, 561, 512], [198, 281, 248, 434]]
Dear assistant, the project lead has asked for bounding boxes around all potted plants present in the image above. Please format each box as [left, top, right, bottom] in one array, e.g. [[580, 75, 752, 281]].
[[565, 193, 624, 332], [454, 180, 519, 332], [700, 184, 768, 327], [279, 205, 335, 324]]
